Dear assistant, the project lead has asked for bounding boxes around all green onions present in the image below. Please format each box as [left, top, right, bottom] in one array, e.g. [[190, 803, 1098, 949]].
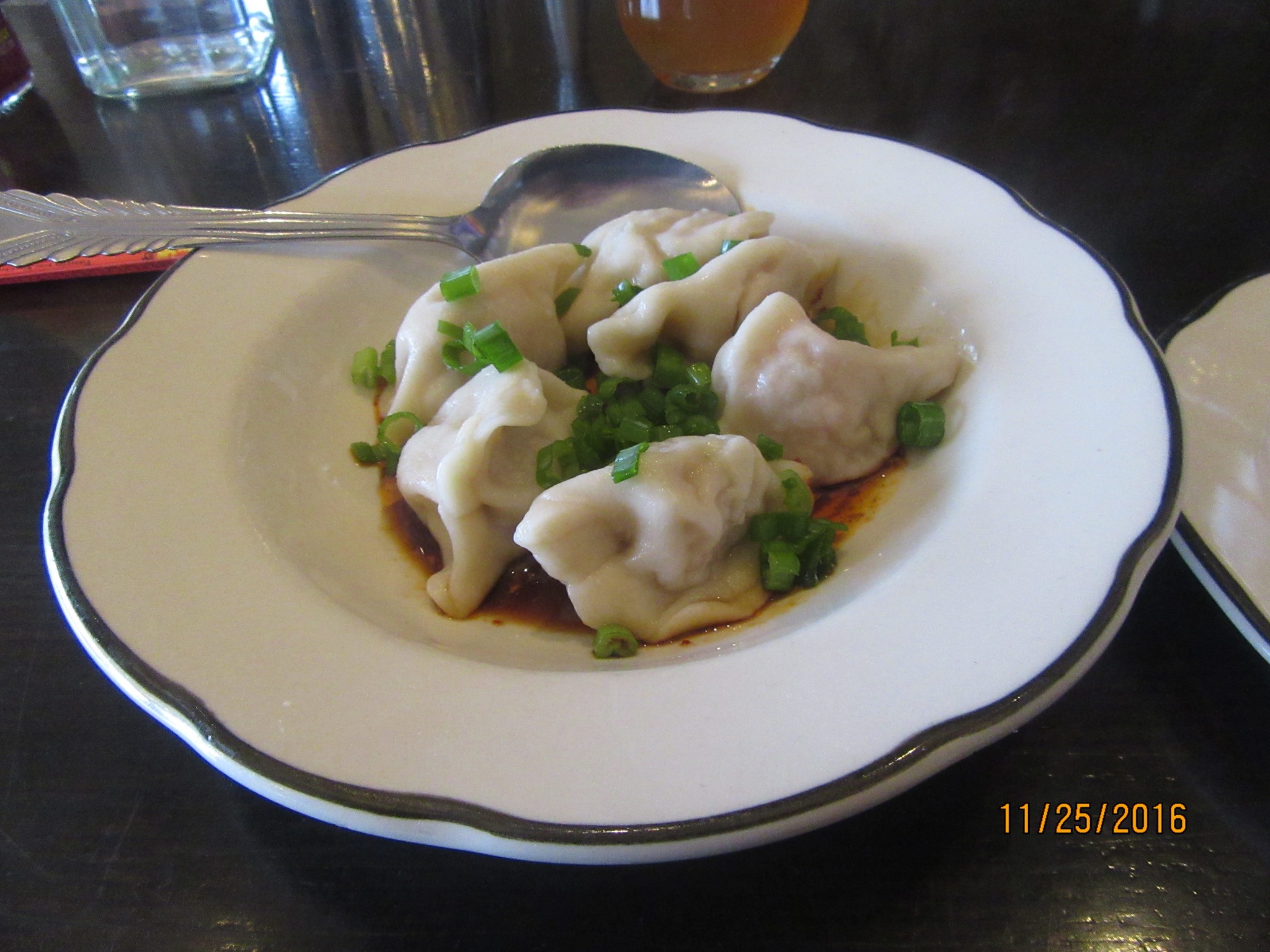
[[342, 239, 945, 660]]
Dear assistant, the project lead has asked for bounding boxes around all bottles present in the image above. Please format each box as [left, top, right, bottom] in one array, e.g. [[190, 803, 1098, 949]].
[[615, 2, 811, 95], [48, 2, 277, 103]]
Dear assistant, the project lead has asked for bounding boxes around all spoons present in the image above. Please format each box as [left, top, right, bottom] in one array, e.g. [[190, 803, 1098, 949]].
[[0, 143, 739, 267]]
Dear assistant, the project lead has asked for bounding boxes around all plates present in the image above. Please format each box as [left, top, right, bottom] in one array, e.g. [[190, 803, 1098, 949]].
[[38, 104, 1268, 868]]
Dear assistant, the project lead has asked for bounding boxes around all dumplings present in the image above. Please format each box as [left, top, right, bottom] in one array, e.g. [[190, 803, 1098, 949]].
[[379, 204, 963, 645]]
